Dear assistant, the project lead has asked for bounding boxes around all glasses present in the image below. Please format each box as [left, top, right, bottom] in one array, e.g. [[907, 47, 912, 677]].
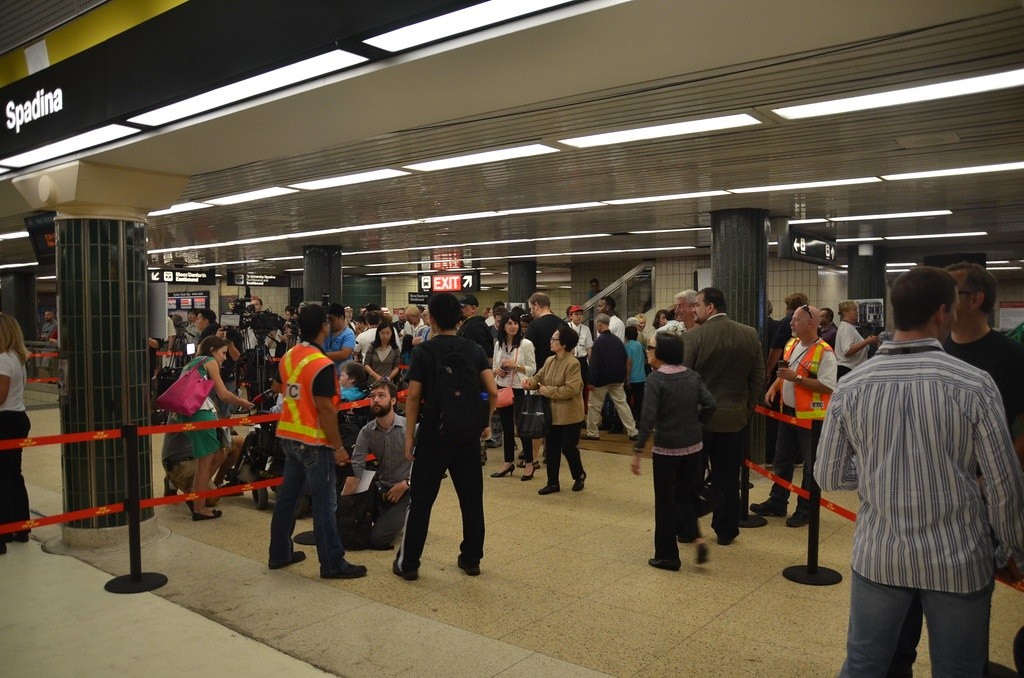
[[802, 304, 812, 319], [551, 338, 559, 342]]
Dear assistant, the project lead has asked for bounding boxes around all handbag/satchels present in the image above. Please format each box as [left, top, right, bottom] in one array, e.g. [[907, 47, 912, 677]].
[[157, 356, 214, 417], [518, 384, 552, 439], [495, 386, 514, 408], [696, 475, 715, 517]]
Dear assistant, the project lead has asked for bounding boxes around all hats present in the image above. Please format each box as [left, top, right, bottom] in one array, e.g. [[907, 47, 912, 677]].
[[459, 295, 478, 307], [569, 305, 584, 315]]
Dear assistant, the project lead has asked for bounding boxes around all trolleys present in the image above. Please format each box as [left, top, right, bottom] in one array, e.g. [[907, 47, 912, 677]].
[[221, 386, 282, 510]]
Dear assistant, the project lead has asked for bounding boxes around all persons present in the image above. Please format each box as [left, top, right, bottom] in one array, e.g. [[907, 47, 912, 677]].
[[0, 261, 1024, 678]]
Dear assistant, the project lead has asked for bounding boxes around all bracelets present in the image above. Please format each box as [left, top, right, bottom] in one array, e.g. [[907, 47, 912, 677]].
[[796, 375, 802, 383], [335, 446, 344, 452], [514, 363, 520, 371]]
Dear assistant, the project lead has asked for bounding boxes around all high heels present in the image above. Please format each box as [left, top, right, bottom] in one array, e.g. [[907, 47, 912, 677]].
[[520, 464, 535, 481], [491, 464, 515, 477]]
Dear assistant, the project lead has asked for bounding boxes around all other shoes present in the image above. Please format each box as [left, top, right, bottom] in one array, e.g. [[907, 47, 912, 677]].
[[321, 558, 367, 578], [193, 509, 222, 520], [697, 544, 706, 564], [164, 476, 177, 496], [538, 485, 560, 494], [269, 551, 306, 568], [581, 434, 600, 440], [393, 562, 418, 580], [458, 555, 480, 575], [629, 435, 638, 441], [649, 559, 681, 570], [572, 471, 587, 491], [786, 507, 810, 527], [750, 500, 789, 516]]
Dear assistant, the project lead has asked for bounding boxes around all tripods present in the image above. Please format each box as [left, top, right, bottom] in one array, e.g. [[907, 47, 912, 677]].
[[236, 331, 278, 413], [167, 333, 188, 368]]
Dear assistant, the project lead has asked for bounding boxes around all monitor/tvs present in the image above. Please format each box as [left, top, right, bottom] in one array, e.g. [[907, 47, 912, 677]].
[[23, 212, 57, 265]]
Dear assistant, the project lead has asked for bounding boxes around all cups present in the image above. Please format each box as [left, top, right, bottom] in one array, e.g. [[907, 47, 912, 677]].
[[777, 360, 789, 369]]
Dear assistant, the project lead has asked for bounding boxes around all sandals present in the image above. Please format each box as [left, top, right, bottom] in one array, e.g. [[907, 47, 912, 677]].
[[516, 458, 540, 469]]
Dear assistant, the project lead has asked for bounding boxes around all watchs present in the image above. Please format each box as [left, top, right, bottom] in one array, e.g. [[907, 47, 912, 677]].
[[405, 479, 411, 485]]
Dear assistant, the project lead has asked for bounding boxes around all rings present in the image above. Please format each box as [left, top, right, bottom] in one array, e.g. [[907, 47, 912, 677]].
[[393, 497, 396, 500]]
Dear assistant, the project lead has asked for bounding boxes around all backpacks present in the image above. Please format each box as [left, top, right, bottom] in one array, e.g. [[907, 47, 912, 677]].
[[415, 339, 481, 443]]
